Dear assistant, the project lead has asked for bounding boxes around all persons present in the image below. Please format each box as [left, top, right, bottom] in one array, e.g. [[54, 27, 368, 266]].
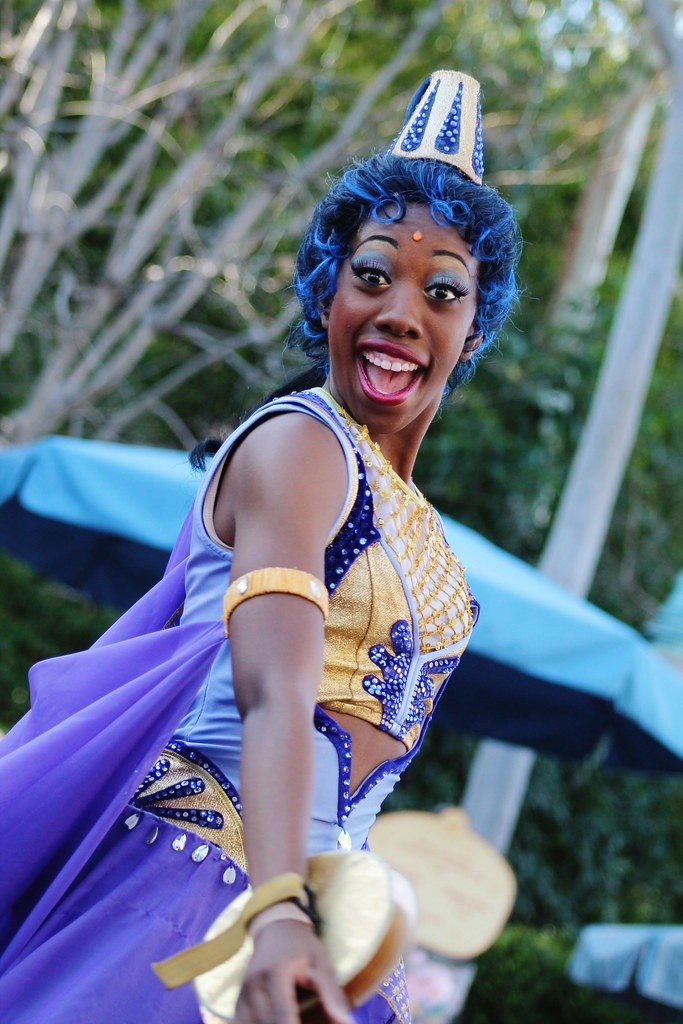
[[0, 71, 519, 1024]]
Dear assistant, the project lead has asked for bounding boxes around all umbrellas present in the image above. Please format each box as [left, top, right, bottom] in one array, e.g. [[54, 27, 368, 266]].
[[0, 436, 683, 776], [565, 922, 683, 1013]]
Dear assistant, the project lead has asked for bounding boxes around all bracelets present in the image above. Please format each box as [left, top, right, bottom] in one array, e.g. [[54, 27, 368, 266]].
[[151, 875, 321, 988]]
